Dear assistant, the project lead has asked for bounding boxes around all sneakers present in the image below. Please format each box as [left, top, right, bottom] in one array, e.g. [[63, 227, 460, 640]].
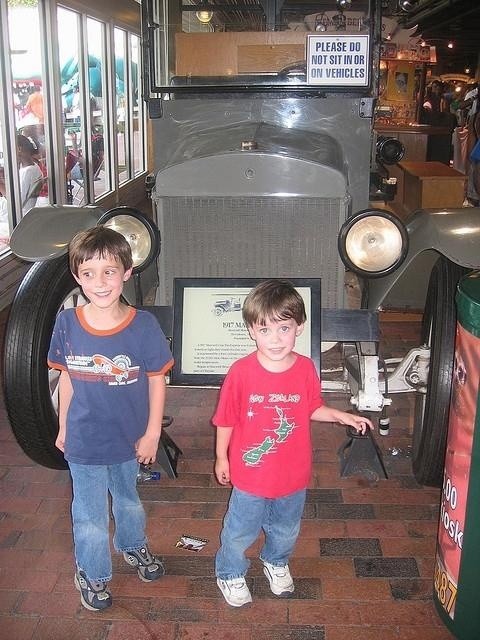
[[263, 562, 294, 598], [217, 576, 252, 608], [74, 571, 113, 611], [123, 544, 165, 583]]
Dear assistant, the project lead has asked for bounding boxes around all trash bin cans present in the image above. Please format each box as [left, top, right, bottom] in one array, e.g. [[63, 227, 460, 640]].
[[431, 273, 480, 640]]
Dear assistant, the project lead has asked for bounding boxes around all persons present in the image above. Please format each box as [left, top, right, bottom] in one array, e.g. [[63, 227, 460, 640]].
[[210, 276, 374, 609], [465, 140, 480, 207], [0, 128, 103, 239], [47, 223, 175, 611]]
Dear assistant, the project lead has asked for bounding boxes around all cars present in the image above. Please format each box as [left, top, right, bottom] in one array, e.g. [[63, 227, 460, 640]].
[[4, 0, 480, 485]]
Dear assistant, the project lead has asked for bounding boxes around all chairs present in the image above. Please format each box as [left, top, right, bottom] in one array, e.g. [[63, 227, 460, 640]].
[[21, 146, 105, 206]]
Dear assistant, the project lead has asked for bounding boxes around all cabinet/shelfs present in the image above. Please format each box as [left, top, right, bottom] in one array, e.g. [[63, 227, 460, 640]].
[[396, 161, 470, 212]]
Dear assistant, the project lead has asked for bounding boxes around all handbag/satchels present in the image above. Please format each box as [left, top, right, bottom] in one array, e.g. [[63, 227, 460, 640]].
[[472, 169, 480, 195]]
